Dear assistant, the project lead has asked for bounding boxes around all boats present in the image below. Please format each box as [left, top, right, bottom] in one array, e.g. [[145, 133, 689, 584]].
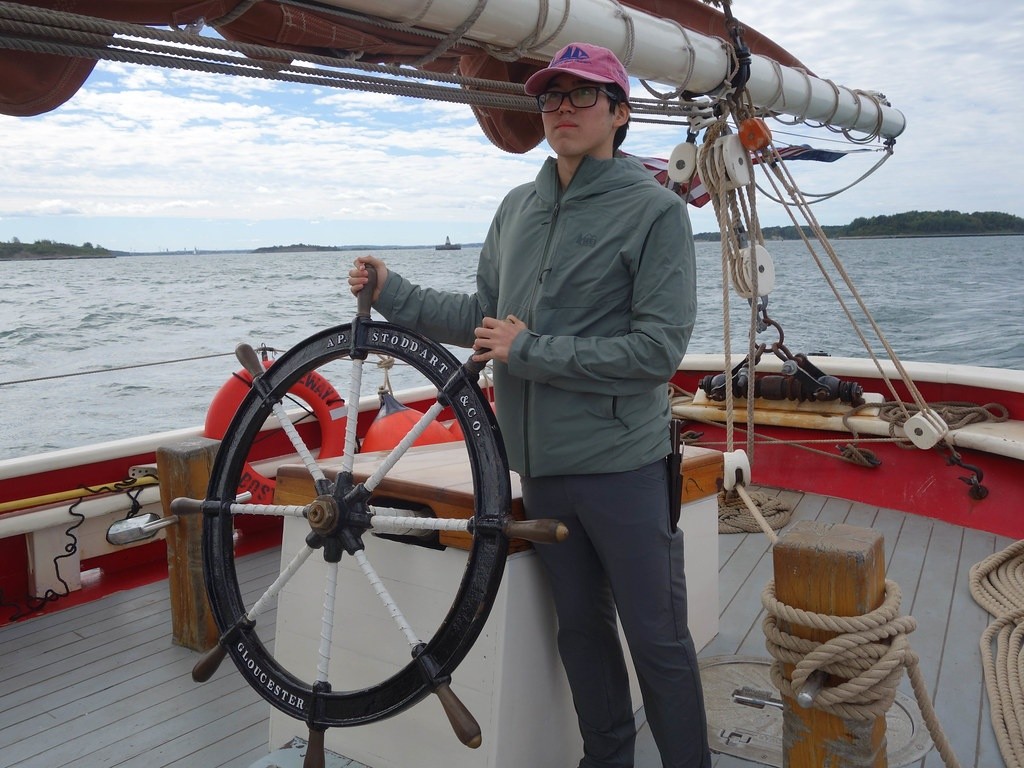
[[434, 236, 461, 250]]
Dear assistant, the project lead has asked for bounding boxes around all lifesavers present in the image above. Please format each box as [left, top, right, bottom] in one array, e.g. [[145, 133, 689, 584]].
[[204, 357, 348, 504]]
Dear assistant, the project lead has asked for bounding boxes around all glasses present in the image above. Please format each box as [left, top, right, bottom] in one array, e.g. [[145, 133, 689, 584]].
[[536, 86, 619, 113]]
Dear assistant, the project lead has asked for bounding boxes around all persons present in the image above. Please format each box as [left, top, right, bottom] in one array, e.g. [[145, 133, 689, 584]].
[[350, 41, 713, 768]]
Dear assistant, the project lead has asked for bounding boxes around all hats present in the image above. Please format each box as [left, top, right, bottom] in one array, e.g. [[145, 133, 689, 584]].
[[524, 43, 630, 101]]
